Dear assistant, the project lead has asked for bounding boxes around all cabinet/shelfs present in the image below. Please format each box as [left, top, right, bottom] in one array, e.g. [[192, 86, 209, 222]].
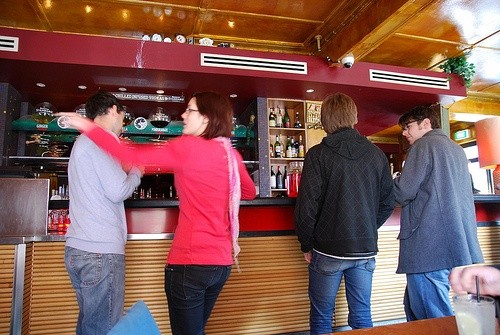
[[267, 97, 308, 191]]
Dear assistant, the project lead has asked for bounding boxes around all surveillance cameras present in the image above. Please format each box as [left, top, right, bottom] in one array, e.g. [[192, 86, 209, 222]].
[[342, 57, 354, 68]]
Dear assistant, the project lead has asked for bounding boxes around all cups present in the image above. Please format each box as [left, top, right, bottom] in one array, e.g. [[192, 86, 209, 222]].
[[452, 294, 496, 335]]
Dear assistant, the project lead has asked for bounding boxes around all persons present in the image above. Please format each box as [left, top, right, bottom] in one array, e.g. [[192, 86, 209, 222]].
[[64, 92, 146, 335], [394, 107, 484, 321], [449, 263, 500, 296], [53, 90, 257, 335], [294, 93, 396, 335]]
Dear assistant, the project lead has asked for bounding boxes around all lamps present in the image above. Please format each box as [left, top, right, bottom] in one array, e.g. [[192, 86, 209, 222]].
[[475, 117, 500, 195]]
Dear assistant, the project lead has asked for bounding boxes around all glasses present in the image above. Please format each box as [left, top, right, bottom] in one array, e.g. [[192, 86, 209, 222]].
[[401, 119, 423, 130], [185, 108, 198, 114]]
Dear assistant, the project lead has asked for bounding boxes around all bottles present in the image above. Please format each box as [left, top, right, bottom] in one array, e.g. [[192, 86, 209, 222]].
[[283, 109, 291, 128], [271, 166, 277, 189], [49, 208, 71, 232], [133, 186, 151, 199], [294, 112, 301, 128], [285, 133, 304, 158], [283, 166, 287, 188], [50, 183, 69, 200], [276, 165, 283, 188], [270, 133, 284, 157], [269, 108, 276, 127], [276, 108, 283, 128], [273, 106, 276, 120]]
